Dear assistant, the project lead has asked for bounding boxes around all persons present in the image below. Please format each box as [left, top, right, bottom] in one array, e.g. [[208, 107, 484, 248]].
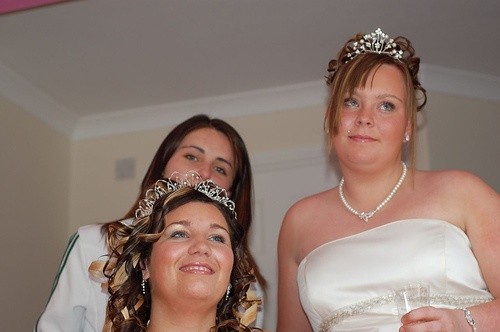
[[31, 112, 273, 331], [87, 168, 267, 331], [275, 26, 500, 332]]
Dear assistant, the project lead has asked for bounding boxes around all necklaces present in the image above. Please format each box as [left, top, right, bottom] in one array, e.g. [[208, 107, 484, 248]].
[[336, 161, 408, 223]]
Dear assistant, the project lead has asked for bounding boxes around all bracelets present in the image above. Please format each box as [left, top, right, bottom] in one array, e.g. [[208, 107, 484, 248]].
[[458, 306, 479, 332]]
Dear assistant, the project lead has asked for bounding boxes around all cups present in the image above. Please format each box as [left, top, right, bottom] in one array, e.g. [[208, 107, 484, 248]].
[[395, 286, 429, 326]]
